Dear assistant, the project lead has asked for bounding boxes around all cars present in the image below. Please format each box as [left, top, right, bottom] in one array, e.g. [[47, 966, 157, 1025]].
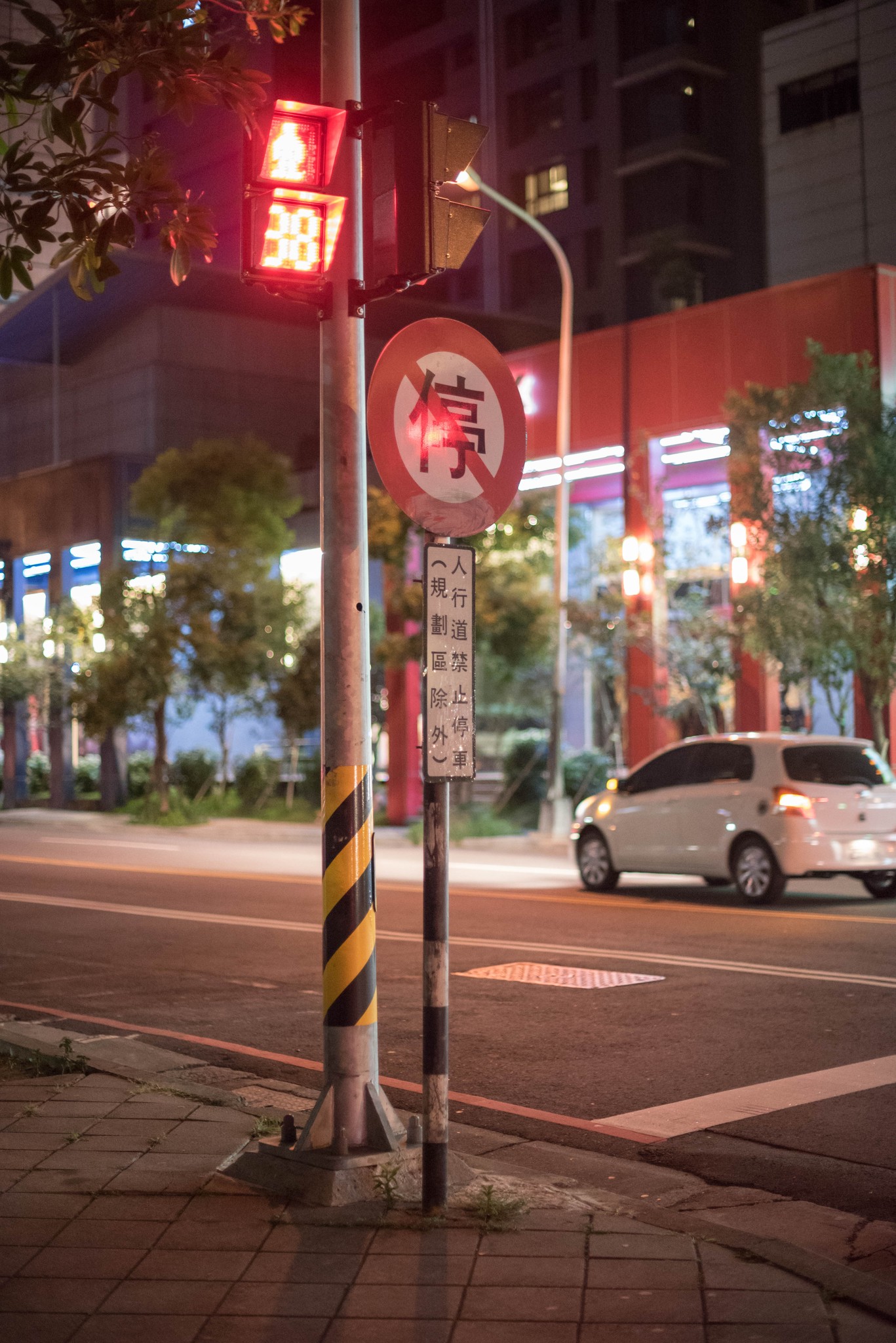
[[572, 731, 896, 904]]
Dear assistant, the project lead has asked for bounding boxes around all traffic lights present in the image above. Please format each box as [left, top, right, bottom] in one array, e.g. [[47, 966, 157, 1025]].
[[363, 95, 494, 286], [241, 89, 356, 295]]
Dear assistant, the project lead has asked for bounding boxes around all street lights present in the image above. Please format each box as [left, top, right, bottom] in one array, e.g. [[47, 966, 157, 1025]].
[[442, 165, 574, 843]]
